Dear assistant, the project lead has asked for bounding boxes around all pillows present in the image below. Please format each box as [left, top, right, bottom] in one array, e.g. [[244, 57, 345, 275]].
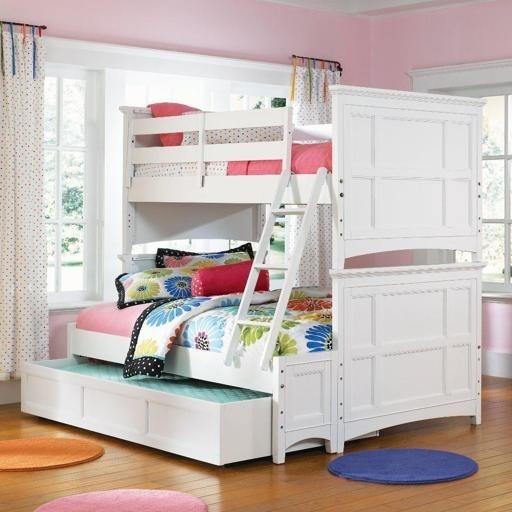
[[146, 103, 296, 147], [115, 243, 295, 309]]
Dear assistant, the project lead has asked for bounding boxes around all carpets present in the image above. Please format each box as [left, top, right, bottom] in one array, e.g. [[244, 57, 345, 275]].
[[326, 447, 478, 485], [0, 438, 104, 472], [34, 489, 208, 512]]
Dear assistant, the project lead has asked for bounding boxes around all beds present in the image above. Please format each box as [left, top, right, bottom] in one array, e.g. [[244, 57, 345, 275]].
[[20, 86, 483, 466]]
